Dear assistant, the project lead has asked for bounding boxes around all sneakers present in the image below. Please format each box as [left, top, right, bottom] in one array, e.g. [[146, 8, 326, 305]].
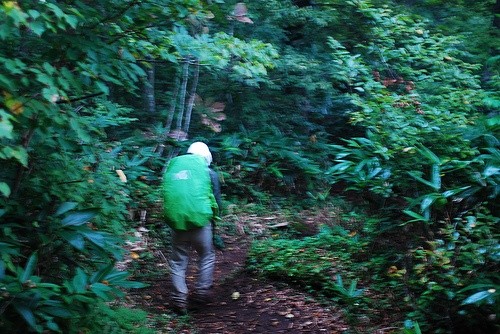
[[170, 300, 187, 316], [191, 286, 218, 303]]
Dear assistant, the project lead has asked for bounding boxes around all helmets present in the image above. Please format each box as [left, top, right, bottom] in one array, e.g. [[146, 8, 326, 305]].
[[186, 141, 213, 166]]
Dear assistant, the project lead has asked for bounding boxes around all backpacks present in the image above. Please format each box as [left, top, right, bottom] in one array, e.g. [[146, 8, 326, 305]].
[[160, 154, 219, 231]]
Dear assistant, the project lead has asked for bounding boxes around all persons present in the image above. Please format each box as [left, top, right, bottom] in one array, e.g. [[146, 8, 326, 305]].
[[161, 141, 222, 316]]
